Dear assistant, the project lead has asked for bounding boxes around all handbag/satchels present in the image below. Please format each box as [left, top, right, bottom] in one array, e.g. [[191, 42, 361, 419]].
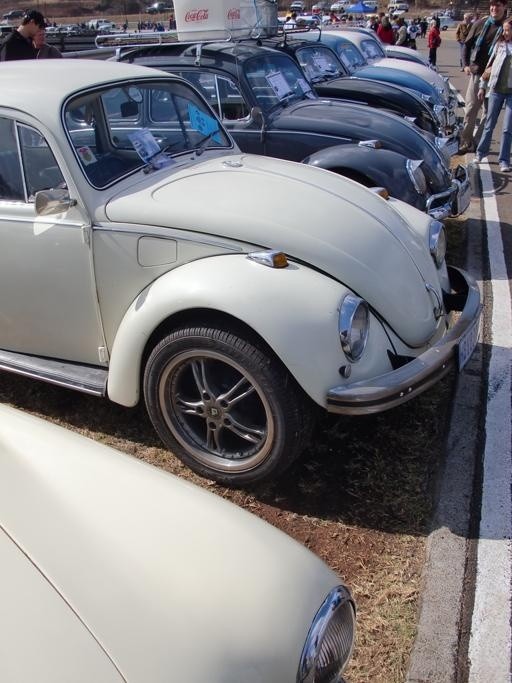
[[469, 64, 484, 75]]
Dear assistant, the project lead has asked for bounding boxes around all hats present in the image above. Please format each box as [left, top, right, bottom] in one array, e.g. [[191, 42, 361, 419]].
[[27, 10, 44, 24]]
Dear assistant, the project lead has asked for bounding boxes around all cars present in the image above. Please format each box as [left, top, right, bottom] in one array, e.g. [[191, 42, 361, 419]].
[[0, 401, 355, 683]]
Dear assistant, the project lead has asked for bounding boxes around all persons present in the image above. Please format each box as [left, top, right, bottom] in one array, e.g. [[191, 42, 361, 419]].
[[472, 16, 512, 171], [112, 15, 176, 34], [456, 11, 474, 69], [31, 24, 65, 57], [456, 0, 506, 154], [1, 7, 43, 64], [283, 8, 442, 70]]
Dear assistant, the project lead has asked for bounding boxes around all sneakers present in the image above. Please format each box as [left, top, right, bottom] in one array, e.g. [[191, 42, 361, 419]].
[[457, 141, 489, 164], [498, 161, 509, 172]]
